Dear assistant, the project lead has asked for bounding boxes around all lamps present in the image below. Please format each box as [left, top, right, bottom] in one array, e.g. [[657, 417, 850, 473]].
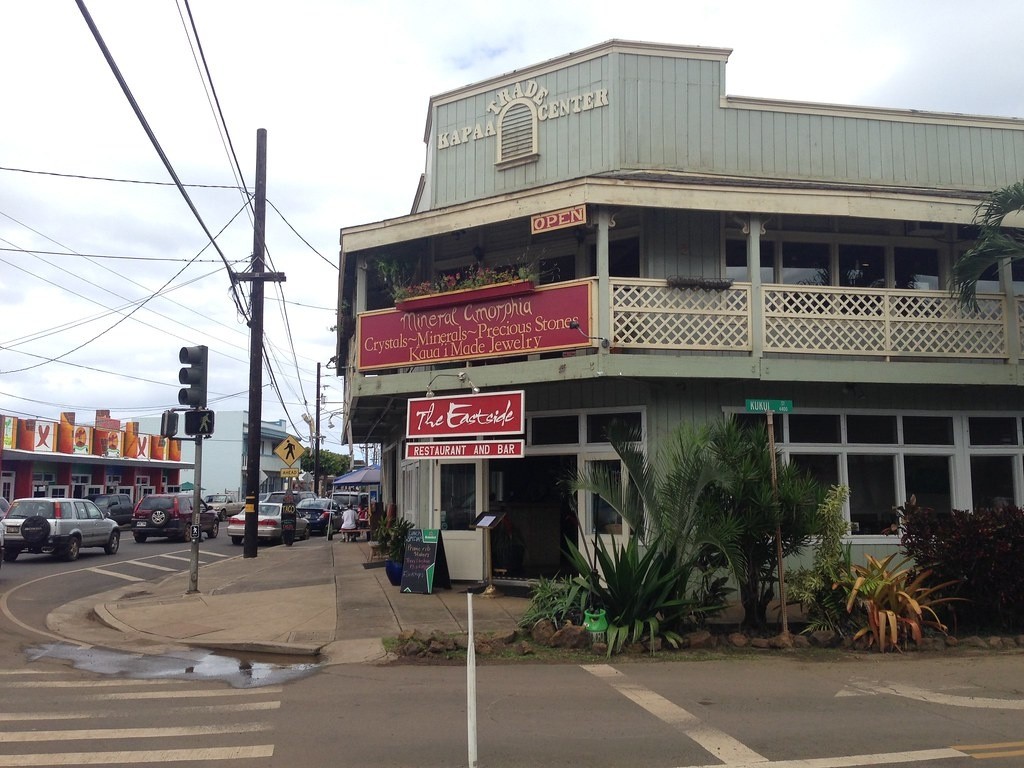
[[472, 244, 483, 261], [573, 226, 585, 247]]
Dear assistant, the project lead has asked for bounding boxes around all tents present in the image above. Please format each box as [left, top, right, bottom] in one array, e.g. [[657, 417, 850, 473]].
[[327, 463, 381, 541]]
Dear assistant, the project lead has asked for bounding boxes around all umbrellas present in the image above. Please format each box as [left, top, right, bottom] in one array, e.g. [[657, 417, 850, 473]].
[[179, 481, 206, 492]]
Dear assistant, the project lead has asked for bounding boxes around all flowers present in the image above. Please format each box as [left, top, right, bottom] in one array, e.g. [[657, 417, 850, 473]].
[[375, 246, 561, 306]]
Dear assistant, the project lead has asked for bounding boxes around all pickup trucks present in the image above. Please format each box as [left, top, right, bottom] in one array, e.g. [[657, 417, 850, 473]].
[[200, 494, 245, 521]]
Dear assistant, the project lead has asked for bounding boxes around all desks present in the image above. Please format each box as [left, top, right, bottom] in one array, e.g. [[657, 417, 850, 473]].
[[358, 519, 368, 529]]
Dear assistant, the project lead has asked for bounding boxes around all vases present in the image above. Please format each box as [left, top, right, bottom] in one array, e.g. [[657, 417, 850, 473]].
[[395, 278, 534, 312]]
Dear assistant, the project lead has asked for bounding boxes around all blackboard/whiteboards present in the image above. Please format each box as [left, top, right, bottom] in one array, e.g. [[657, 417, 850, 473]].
[[400, 528, 439, 594]]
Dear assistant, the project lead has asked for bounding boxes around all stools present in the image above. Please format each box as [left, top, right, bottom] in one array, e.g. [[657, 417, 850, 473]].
[[366, 541, 391, 563]]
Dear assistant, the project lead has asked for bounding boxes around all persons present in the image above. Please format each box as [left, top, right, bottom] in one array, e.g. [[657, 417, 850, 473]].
[[341, 504, 361, 542]]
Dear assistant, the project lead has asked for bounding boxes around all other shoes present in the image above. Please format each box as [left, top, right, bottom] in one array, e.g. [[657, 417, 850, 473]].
[[351, 538, 357, 542], [340, 538, 345, 542]]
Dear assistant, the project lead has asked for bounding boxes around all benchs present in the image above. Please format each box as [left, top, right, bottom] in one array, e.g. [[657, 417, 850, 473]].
[[339, 529, 378, 543]]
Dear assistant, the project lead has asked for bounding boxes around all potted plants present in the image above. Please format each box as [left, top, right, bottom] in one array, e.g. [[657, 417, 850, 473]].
[[370, 515, 415, 586]]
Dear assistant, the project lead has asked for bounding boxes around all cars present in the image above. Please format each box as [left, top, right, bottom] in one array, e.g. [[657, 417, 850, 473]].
[[227, 490, 369, 547]]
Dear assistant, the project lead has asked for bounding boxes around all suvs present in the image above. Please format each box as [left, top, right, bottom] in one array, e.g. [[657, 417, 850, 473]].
[[131, 493, 219, 543], [0, 497, 121, 562], [82, 494, 134, 526]]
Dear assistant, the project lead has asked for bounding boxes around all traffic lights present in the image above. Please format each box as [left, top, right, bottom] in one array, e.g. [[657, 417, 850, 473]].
[[185, 410, 214, 435], [178, 345, 208, 407]]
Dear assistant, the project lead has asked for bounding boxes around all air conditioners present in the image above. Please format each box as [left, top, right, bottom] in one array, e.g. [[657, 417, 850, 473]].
[[905, 221, 945, 236]]
